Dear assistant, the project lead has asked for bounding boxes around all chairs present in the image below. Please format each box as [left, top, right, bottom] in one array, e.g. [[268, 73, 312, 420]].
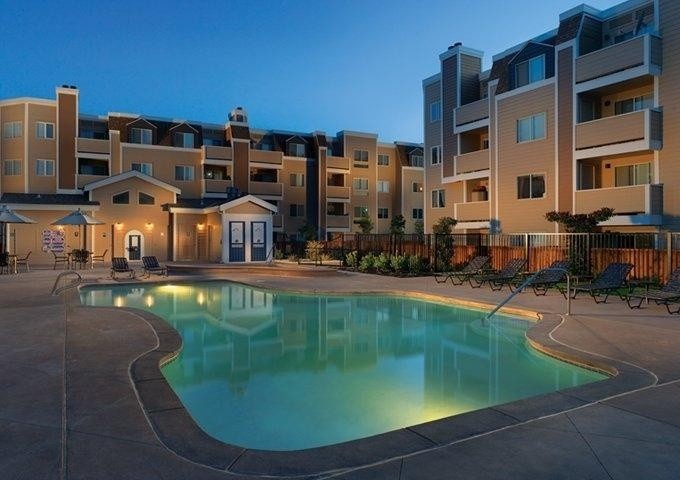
[[0, 249, 168, 280], [432, 254, 679, 315]]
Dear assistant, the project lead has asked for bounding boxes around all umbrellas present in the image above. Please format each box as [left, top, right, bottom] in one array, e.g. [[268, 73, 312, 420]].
[[50, 207, 105, 252], [0, 205, 38, 253]]
